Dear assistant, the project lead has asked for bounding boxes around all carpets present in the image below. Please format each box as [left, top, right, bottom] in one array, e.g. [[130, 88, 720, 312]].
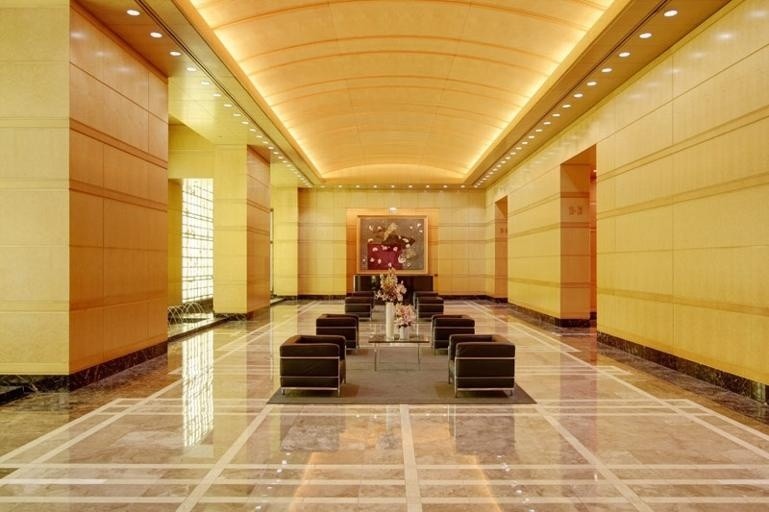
[[265, 346, 538, 405]]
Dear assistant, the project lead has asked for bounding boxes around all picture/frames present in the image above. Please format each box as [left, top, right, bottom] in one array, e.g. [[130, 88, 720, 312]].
[[355, 213, 429, 275]]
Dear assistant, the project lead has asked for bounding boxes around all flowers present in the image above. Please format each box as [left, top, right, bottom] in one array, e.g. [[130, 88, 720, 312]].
[[376, 268, 407, 302], [395, 304, 415, 327]]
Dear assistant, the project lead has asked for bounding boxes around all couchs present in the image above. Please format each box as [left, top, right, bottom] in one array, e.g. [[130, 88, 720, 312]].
[[344, 290, 444, 322], [430, 314, 476, 354], [447, 335, 517, 396], [316, 313, 359, 350], [278, 335, 347, 397]]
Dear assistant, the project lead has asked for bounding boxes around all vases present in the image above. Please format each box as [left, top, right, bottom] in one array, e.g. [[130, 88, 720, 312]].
[[386, 302, 395, 340], [400, 326, 410, 340]]
